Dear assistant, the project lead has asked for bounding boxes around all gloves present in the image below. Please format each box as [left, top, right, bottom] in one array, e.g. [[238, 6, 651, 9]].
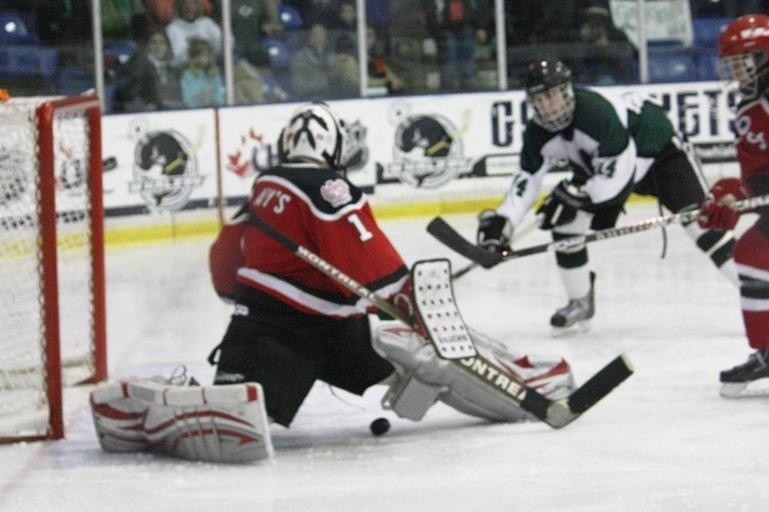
[[535, 178, 586, 229], [477, 213, 518, 257]]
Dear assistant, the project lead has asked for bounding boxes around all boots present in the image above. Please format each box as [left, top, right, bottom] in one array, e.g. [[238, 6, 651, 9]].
[[715, 349, 769, 398], [548, 272, 595, 338]]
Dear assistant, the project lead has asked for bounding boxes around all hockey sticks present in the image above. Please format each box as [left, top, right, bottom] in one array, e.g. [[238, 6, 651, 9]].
[[245, 212, 633, 430], [427, 194, 769, 269]]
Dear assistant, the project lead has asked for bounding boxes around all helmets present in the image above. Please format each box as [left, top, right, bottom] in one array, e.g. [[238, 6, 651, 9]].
[[713, 13, 768, 104], [271, 3, 304, 34], [260, 36, 293, 71], [275, 99, 370, 173], [522, 55, 578, 132]]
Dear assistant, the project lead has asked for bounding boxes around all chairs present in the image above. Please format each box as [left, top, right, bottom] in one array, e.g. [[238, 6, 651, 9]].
[[629, 14, 735, 87]]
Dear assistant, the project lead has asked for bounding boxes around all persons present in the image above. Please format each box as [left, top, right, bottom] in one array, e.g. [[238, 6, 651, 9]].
[[693, 13, 769, 385], [85, 99, 577, 465], [100, 0, 361, 113], [334, 0, 498, 94], [505, 1, 638, 89], [474, 54, 743, 330]]
